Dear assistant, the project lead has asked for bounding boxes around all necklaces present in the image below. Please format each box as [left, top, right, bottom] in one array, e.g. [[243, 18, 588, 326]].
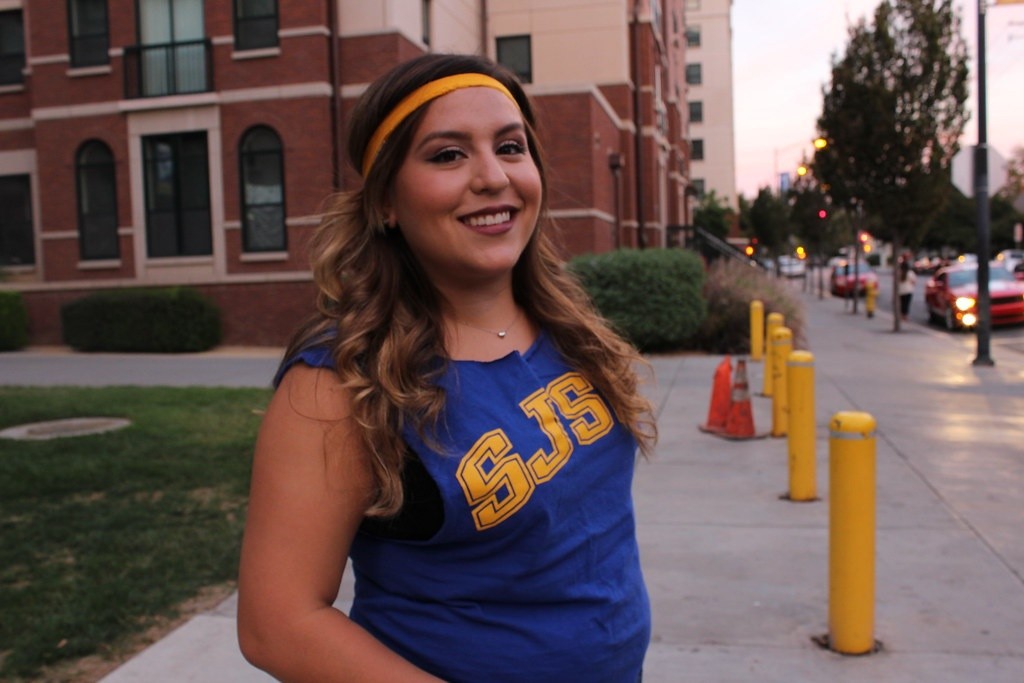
[[443, 310, 520, 338]]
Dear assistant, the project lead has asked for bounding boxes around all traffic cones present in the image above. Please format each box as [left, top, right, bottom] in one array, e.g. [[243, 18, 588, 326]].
[[716, 357, 765, 443], [697, 356, 732, 435]]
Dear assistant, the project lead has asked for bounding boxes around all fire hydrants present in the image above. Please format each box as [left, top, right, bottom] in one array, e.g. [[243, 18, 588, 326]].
[[867, 281, 878, 316]]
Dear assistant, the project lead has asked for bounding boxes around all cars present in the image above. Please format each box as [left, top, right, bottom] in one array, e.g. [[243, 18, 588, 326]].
[[831, 262, 879, 298], [996, 250, 1024, 281], [925, 262, 1024, 332]]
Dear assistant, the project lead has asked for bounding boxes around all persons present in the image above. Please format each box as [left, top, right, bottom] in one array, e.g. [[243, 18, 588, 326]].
[[236, 54, 658, 683], [899, 260, 917, 322]]
